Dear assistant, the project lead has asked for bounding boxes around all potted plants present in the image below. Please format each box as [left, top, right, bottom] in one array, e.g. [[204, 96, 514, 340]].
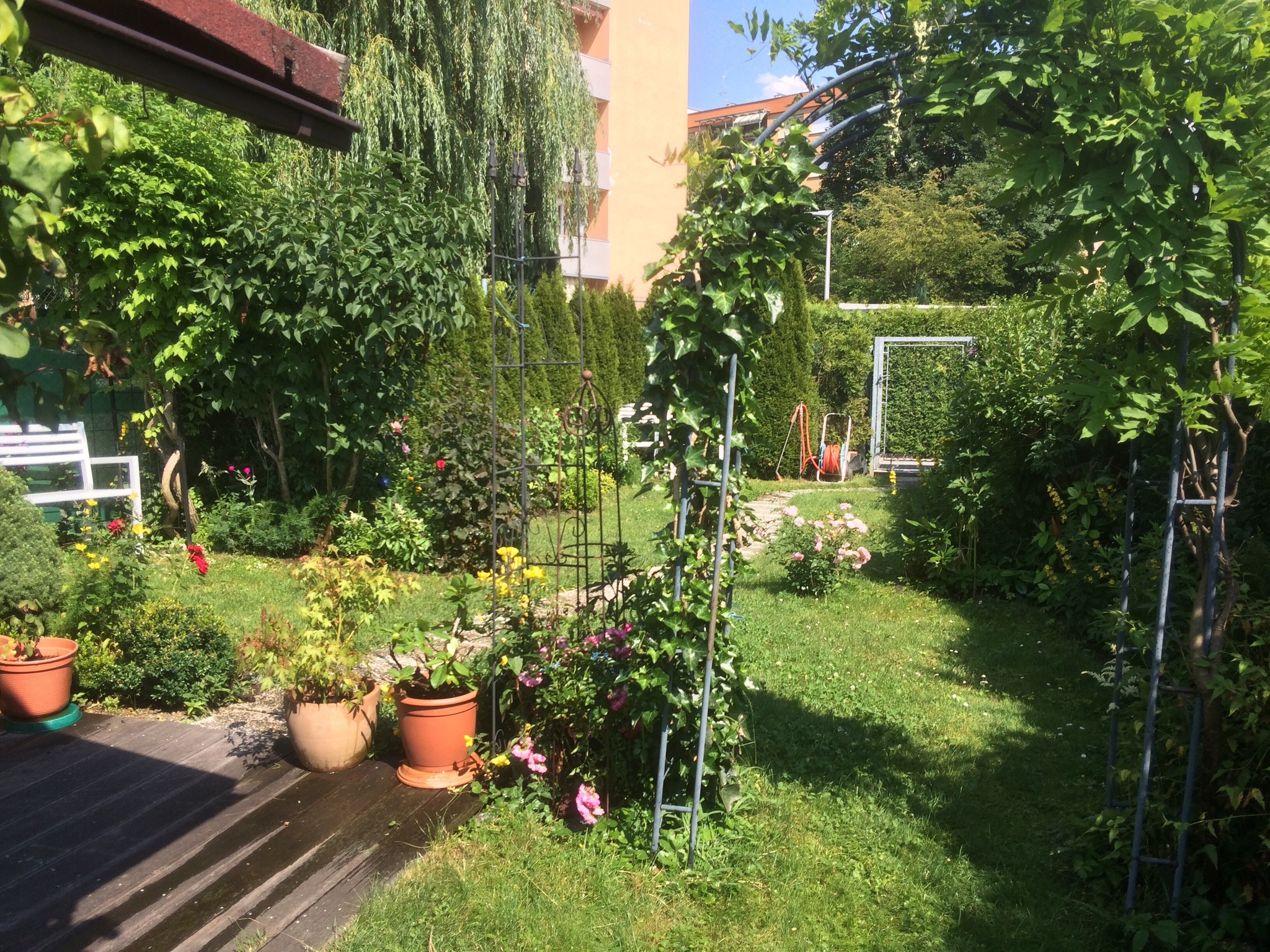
[[370, 569, 486, 788], [240, 545, 420, 771], [0, 599, 78, 717]]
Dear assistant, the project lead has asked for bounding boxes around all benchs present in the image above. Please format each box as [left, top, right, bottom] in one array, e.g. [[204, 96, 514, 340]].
[[619, 400, 724, 467], [0, 422, 145, 555]]
